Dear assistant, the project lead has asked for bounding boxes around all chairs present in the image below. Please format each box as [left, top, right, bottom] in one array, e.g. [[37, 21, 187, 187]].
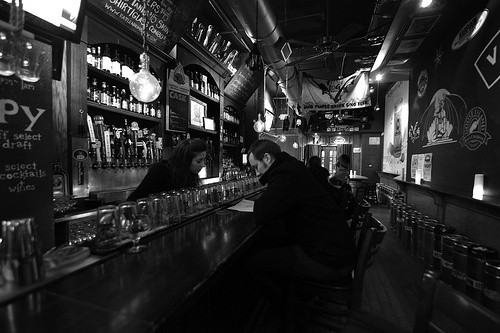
[[297, 199, 388, 322]]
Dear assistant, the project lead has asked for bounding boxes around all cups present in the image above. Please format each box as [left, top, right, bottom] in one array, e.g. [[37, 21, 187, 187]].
[[0, 158, 265, 290]]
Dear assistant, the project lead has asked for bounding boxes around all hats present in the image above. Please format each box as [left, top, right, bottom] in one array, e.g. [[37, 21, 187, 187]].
[[337, 154, 350, 164]]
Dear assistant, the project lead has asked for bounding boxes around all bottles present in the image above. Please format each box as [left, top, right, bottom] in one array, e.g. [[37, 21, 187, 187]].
[[85, 42, 244, 163]]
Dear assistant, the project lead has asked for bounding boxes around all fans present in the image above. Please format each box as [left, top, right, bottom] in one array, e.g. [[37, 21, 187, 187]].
[[283, 0, 384, 74]]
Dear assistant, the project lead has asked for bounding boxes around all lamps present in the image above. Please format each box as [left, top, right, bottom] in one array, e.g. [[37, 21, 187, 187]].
[[130, 0, 162, 103]]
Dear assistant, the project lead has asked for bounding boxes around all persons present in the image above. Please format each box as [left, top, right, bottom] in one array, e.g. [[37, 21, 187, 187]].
[[305, 156, 330, 184], [330, 154, 351, 187], [127, 137, 208, 201], [246, 140, 358, 285]]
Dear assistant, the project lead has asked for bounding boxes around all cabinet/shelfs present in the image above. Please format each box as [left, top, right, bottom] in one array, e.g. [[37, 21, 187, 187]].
[[221, 117, 245, 180], [86, 62, 163, 169], [166, 86, 224, 183]]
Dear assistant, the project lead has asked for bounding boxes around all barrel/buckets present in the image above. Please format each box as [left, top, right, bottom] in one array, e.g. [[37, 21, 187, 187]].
[[376, 184, 500, 314]]
